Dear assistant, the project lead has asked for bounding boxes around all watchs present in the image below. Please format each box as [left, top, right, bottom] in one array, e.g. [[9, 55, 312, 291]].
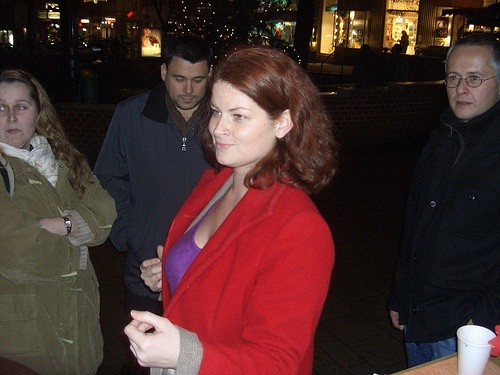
[[63, 217, 72, 236]]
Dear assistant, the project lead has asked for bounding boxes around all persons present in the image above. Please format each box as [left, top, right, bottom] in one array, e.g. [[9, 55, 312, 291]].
[[398, 30, 409, 53], [123, 47, 339, 375], [0, 67, 117, 375], [388, 33, 500, 367], [92, 35, 213, 315]]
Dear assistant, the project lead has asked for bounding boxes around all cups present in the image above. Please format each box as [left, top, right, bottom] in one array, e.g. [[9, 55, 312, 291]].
[[456, 324, 496, 375]]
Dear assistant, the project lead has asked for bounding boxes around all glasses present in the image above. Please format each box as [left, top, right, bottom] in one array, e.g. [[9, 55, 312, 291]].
[[442, 75, 498, 88]]
